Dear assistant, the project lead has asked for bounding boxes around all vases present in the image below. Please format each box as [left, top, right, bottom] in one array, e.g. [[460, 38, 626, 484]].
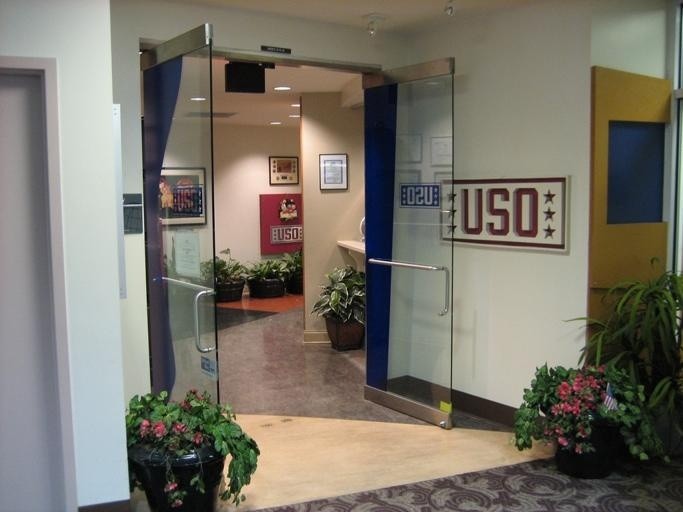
[[129, 447, 225, 511], [555, 450, 592, 474]]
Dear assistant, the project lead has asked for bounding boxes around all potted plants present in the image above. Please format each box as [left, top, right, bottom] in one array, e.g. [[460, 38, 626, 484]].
[[309, 267, 365, 350], [197, 246, 303, 302]]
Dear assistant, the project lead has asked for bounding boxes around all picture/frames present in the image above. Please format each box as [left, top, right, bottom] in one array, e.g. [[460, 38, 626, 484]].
[[268, 156, 298, 186], [159, 167, 206, 227], [318, 154, 347, 190]]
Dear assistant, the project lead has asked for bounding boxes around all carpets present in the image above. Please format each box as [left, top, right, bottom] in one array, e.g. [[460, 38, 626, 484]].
[[256, 451, 683, 511]]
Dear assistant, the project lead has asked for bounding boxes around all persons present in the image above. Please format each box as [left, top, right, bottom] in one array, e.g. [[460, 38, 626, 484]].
[[171, 190, 193, 213], [158, 176, 177, 209], [173, 176, 194, 201]]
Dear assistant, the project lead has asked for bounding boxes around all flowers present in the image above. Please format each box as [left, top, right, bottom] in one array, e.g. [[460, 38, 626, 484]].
[[512, 362, 668, 463], [124, 388, 257, 506]]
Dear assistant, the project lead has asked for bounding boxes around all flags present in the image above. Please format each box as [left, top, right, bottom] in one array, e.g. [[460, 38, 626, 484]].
[[604, 382, 618, 412]]
